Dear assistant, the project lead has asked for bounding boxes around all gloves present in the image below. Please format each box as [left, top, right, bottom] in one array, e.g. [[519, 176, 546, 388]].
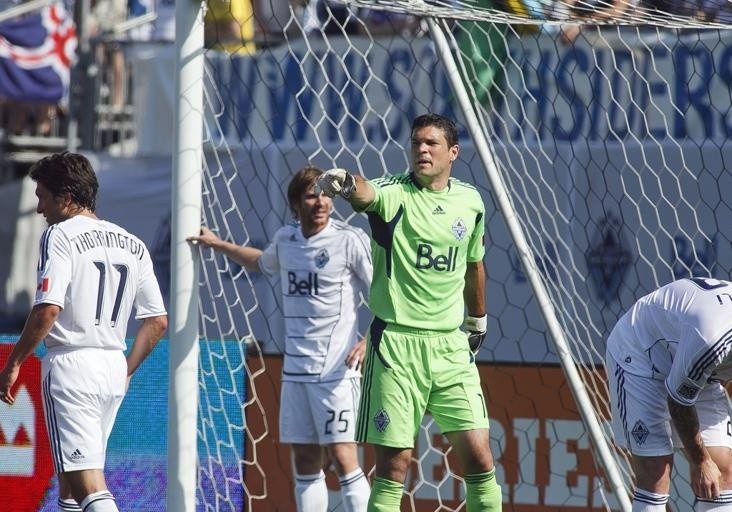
[[317, 167, 357, 203], [463, 313, 488, 358]]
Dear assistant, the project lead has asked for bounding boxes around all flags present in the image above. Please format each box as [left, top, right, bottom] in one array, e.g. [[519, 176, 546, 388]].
[[1, 0, 77, 102]]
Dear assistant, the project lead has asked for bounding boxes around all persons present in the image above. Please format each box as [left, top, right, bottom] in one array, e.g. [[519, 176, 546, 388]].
[[313, 114, 501, 510], [600, 277, 730, 511], [1, 152, 172, 509], [184, 167, 373, 509]]
